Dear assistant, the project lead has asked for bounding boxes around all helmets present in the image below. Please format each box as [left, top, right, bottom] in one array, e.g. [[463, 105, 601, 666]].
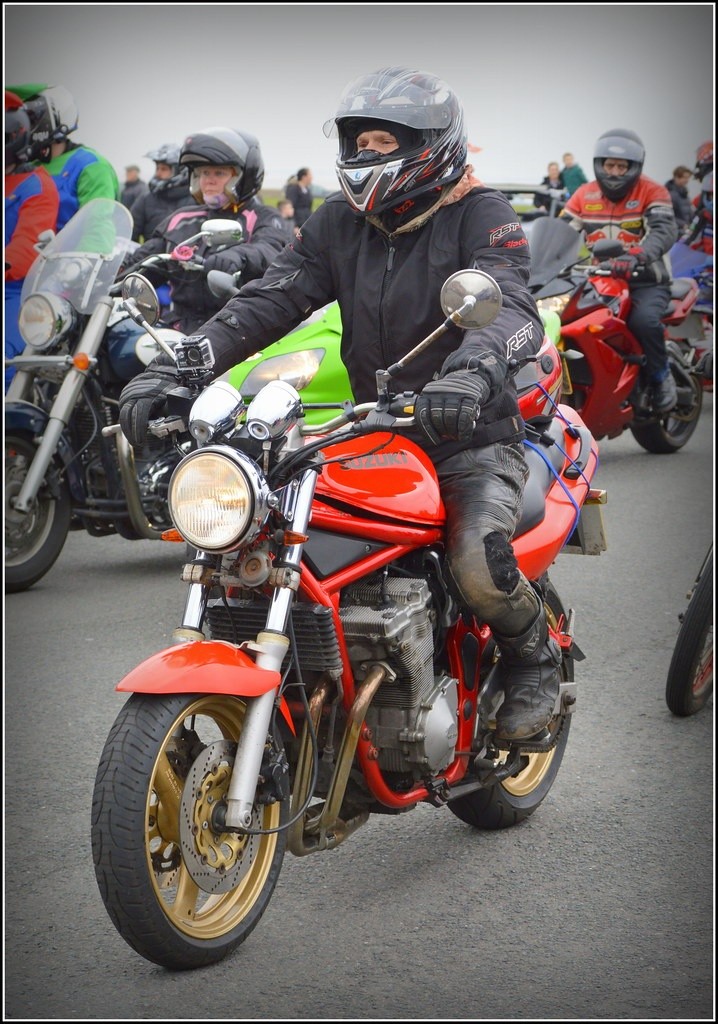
[[5, 91, 32, 167], [177, 127, 264, 215], [323, 68, 466, 217], [594, 128, 644, 201], [145, 143, 190, 196], [5, 84, 79, 153], [690, 142, 713, 192]]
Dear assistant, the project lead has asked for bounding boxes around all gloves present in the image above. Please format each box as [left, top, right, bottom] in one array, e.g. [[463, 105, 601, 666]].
[[114, 343, 186, 447], [204, 245, 246, 276], [411, 367, 493, 448], [610, 246, 647, 281]]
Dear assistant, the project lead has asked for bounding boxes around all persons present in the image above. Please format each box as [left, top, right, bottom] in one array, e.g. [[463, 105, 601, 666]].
[[534, 128, 713, 413], [117, 64, 563, 741], [5, 82, 313, 392]]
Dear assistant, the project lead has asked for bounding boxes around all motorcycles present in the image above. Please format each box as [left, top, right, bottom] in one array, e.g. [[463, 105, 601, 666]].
[[522, 216, 713, 454], [6, 198, 246, 592], [89, 268, 606, 972], [665, 542, 712, 718]]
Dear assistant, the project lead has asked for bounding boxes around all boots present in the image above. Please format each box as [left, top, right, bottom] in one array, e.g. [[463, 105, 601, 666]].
[[649, 361, 677, 413], [489, 581, 562, 741]]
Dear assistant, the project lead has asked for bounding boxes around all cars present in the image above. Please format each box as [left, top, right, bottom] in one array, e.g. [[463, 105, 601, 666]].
[[215, 266, 564, 428]]
